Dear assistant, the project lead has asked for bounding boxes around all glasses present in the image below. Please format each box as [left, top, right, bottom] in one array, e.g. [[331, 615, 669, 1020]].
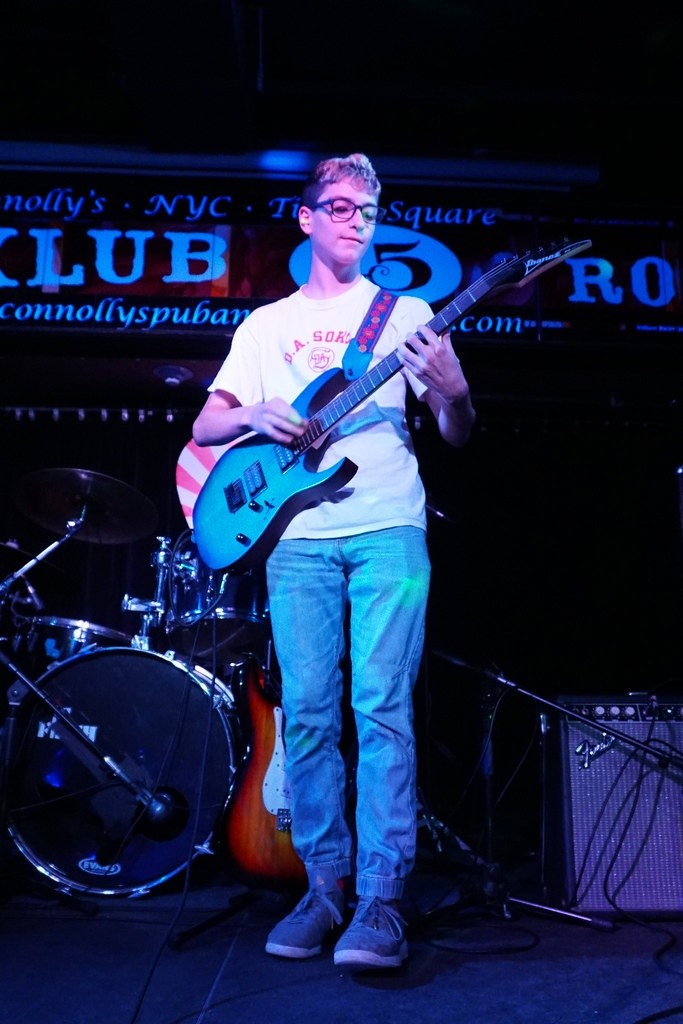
[[308, 197, 388, 227]]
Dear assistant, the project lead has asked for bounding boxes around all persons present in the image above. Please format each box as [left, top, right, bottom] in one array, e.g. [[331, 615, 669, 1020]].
[[193, 153, 475, 967]]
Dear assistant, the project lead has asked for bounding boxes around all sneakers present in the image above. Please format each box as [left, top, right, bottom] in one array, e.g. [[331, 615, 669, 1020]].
[[332, 897, 409, 971], [265, 889, 356, 961]]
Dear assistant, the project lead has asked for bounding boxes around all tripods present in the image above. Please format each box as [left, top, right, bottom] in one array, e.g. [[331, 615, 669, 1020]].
[[422, 651, 683, 931]]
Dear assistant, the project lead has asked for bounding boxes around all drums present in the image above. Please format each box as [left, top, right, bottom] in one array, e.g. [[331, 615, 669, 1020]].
[[26, 612, 127, 687], [0, 646, 246, 900], [162, 537, 269, 659]]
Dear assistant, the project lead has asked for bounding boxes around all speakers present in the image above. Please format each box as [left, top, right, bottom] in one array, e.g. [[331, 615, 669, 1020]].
[[551, 701, 683, 914]]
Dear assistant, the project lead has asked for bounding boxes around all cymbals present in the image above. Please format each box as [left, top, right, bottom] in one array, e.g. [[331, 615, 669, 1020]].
[[0, 465, 159, 544]]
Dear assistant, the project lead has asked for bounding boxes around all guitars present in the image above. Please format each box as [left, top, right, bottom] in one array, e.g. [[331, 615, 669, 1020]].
[[213, 645, 308, 888], [190, 232, 592, 575]]
